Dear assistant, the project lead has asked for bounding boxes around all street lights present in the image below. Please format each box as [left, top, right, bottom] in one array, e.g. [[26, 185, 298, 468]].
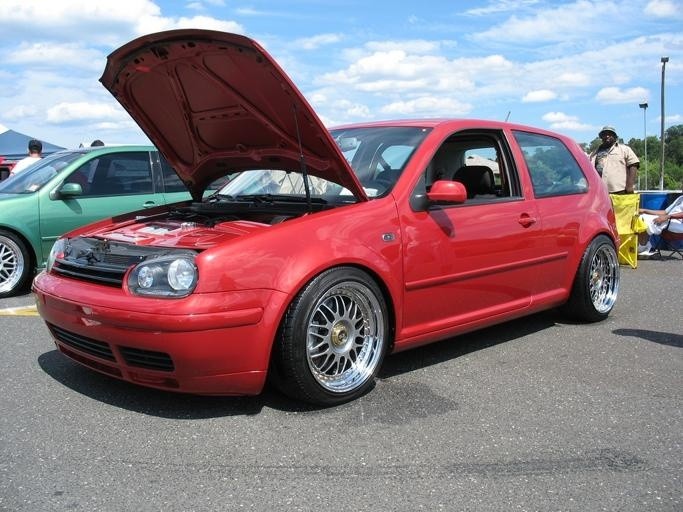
[[638, 98, 648, 191], [658, 55, 669, 190]]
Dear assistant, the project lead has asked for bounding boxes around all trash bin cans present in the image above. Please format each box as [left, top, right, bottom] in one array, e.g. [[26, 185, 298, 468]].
[[641, 193, 668, 210]]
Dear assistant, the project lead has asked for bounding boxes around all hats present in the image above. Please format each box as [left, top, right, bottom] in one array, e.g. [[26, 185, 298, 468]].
[[29, 139, 43, 150], [599, 127, 617, 138]]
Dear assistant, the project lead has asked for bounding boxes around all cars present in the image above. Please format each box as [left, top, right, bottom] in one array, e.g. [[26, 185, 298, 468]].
[[31, 28, 620, 408], [0, 146, 231, 300]]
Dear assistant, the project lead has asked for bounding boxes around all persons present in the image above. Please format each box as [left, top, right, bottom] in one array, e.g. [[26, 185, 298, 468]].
[[9, 139, 58, 193], [636, 193, 682, 257], [587, 125, 641, 196], [86, 139, 116, 184]]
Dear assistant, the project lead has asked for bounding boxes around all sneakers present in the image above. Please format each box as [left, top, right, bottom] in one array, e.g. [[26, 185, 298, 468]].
[[638, 241, 650, 253], [638, 248, 659, 255]]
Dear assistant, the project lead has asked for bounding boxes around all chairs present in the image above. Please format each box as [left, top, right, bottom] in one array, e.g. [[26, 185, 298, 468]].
[[375, 166, 501, 199], [609, 193, 683, 269]]
